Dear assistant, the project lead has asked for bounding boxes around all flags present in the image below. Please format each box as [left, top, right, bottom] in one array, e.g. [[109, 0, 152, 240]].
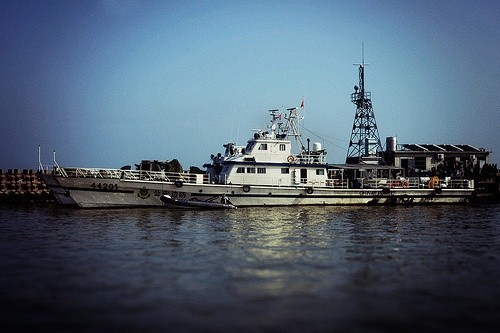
[[301, 101, 304, 108], [279, 114, 282, 120]]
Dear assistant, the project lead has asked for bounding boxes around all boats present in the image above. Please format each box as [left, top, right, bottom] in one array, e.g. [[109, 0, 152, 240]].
[[34, 101, 475, 210], [160, 194, 235, 211]]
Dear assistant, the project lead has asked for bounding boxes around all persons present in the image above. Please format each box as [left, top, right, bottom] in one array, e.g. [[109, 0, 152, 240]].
[[221, 193, 234, 206]]
